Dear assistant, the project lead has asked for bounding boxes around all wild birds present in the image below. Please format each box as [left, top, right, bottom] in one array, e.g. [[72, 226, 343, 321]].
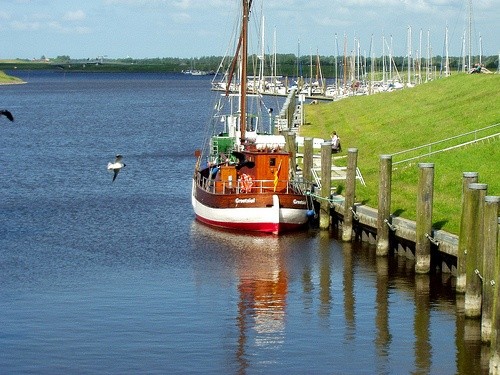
[[107, 153, 129, 184], [0, 109, 16, 123], [229, 150, 257, 171]]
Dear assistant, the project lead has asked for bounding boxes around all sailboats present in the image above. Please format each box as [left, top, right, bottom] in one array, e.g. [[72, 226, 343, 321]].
[[181, 0, 493, 122], [191, 0, 314, 235]]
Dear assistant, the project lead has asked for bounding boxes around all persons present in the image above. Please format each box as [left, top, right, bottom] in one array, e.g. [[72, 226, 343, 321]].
[[330, 130, 342, 155], [470, 63, 482, 74]]
